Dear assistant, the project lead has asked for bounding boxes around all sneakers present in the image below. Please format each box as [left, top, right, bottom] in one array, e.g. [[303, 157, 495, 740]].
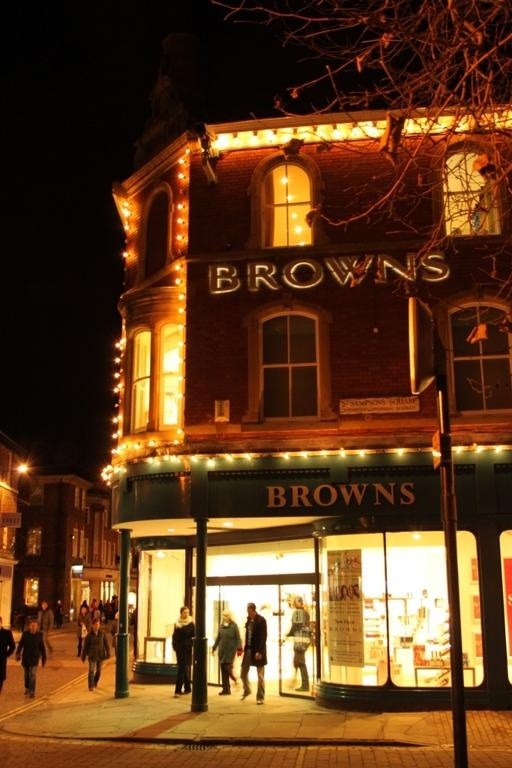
[[174, 691, 181, 698], [256, 696, 264, 705], [88, 683, 98, 692], [23, 689, 36, 699], [240, 691, 252, 701], [218, 691, 232, 695], [181, 690, 191, 695]]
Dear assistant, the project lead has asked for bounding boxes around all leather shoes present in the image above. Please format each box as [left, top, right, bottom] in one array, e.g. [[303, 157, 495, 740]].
[[295, 686, 310, 692]]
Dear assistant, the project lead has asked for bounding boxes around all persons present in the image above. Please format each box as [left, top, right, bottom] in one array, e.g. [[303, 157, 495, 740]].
[[210, 609, 244, 696], [280, 594, 315, 692], [329, 583, 360, 601], [0, 589, 120, 700], [239, 602, 269, 705], [171, 604, 196, 699]]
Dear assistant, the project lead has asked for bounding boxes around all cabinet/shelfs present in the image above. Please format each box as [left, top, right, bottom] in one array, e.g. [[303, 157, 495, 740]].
[[414, 666, 476, 688], [144, 636, 173, 664], [362, 594, 410, 665]]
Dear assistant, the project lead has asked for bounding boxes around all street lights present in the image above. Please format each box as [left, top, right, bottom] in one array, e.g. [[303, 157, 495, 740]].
[[407, 294, 468, 768]]
